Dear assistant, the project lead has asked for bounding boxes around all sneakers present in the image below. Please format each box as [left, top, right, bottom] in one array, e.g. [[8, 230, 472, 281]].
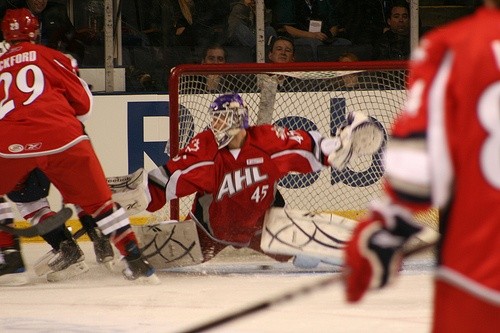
[[48, 241, 83, 270], [0, 252, 25, 276], [122, 260, 153, 280], [93, 239, 115, 262]]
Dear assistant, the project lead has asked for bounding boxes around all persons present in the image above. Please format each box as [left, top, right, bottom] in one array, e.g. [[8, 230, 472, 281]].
[[0, 0, 411, 282], [341, 1, 500, 333]]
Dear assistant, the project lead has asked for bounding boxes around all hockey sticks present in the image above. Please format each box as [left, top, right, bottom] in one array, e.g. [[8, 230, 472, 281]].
[[33, 220, 96, 280], [0, 207, 72, 238]]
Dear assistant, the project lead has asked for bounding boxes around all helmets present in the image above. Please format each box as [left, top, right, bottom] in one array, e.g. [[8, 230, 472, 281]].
[[2, 8, 38, 40], [208, 93, 249, 149]]
